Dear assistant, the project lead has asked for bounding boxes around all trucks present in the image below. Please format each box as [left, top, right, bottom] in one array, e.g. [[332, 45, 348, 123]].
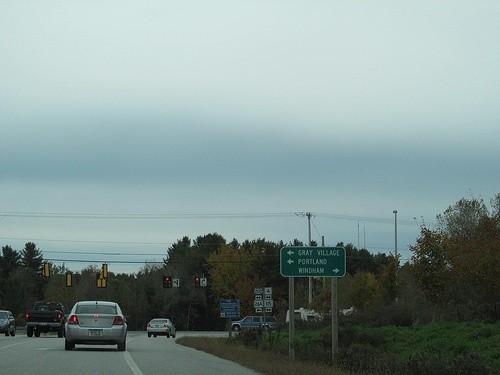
[[285, 308, 322, 323]]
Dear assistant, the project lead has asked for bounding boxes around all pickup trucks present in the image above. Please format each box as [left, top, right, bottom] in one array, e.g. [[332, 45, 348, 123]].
[[25, 301, 67, 338]]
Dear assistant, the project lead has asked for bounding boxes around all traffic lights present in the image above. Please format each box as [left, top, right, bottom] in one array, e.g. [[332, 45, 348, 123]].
[[163, 276, 172, 288], [195, 276, 200, 288]]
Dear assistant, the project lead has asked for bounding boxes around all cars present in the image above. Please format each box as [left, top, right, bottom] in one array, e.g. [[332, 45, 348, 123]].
[[65, 301, 128, 351], [0, 310, 16, 337], [229, 315, 280, 332], [147, 318, 176, 338]]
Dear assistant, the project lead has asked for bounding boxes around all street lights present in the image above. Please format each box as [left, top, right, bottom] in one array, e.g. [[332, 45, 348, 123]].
[[133, 275, 138, 331], [392, 210, 399, 303]]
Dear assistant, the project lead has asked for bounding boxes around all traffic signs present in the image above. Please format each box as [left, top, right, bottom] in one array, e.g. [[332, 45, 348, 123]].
[[279, 246, 347, 278]]
